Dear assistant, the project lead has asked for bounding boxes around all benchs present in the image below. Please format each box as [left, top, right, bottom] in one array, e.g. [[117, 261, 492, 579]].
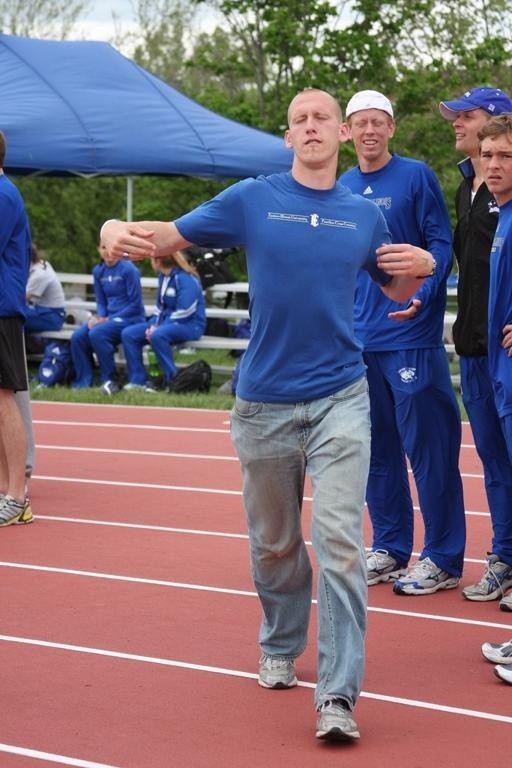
[[45, 271, 253, 351]]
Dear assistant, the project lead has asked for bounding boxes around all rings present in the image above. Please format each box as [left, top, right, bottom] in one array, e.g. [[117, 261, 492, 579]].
[[123, 252, 129, 261]]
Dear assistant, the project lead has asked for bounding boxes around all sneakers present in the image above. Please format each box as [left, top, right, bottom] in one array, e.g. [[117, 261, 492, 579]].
[[146, 382, 170, 394], [480, 639, 512, 665], [494, 664, 512, 684], [258, 655, 299, 689], [462, 554, 512, 601], [393, 557, 461, 596], [315, 699, 360, 742], [124, 381, 149, 391], [499, 593, 512, 612], [101, 381, 122, 396], [0, 495, 35, 527], [366, 549, 409, 587]]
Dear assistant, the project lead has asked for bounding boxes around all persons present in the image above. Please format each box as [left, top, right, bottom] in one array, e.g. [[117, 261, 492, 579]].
[[121, 250, 206, 393], [70, 239, 146, 395], [439, 87, 512, 612], [0, 131, 34, 527], [100, 89, 437, 740], [477, 112, 512, 684], [25, 245, 66, 331], [337, 89, 466, 596]]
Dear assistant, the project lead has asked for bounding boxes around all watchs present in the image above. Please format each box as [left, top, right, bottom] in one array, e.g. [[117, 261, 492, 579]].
[[416, 259, 437, 279]]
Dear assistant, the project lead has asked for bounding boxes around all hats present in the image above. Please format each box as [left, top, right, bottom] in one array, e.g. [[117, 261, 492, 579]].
[[346, 91, 393, 119], [437, 88, 511, 125]]
